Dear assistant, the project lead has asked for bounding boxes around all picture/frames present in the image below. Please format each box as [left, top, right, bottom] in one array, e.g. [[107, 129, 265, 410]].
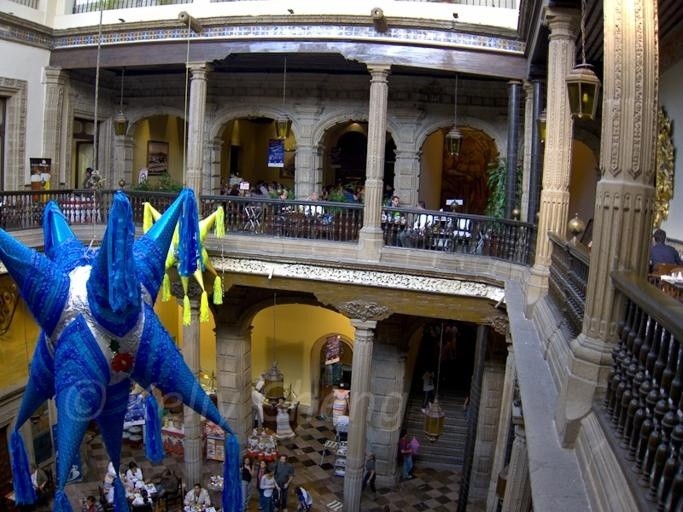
[[146, 140, 169, 176]]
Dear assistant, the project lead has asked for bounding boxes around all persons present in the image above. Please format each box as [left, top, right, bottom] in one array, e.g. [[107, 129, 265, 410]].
[[444, 324, 460, 361], [82, 167, 95, 196], [84, 377, 350, 512], [398, 429, 415, 479], [463, 387, 471, 424], [421, 367, 436, 415], [303, 180, 365, 218], [399, 201, 436, 248], [385, 197, 401, 219], [220, 169, 294, 214], [28, 464, 51, 506], [384, 185, 393, 197], [362, 451, 378, 501], [650, 229, 680, 271]]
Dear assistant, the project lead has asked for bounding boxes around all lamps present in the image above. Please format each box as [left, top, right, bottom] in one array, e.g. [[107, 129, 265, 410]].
[[537, 1, 600, 144], [112, 66, 129, 137], [445, 71, 464, 159], [272, 66, 292, 139], [262, 289, 283, 408], [423, 317, 447, 443]]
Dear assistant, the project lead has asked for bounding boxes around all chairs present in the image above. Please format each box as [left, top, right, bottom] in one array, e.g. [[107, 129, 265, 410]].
[[96, 460, 183, 512]]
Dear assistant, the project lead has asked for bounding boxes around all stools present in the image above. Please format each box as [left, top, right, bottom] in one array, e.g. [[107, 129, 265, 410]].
[[319, 438, 337, 470]]
